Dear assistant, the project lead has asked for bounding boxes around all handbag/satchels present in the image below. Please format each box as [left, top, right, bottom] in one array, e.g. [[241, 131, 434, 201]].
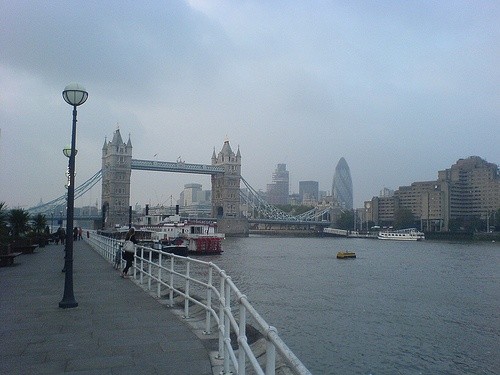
[[123, 235, 134, 253]]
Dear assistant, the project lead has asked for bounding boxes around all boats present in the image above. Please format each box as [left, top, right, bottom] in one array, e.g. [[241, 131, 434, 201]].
[[97, 217, 226, 256], [377, 227, 426, 241], [336, 249, 357, 259]]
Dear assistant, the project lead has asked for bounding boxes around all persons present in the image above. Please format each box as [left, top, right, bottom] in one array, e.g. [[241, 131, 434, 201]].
[[73, 227, 78, 241], [59, 228, 65, 245], [119, 228, 138, 279], [78, 227, 83, 240]]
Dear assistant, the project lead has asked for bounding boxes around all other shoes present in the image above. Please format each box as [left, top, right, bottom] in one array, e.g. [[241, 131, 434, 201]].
[[120, 272, 131, 279]]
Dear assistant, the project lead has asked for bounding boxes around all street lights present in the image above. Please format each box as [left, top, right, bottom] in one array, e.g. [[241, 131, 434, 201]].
[[58, 82, 89, 309]]
